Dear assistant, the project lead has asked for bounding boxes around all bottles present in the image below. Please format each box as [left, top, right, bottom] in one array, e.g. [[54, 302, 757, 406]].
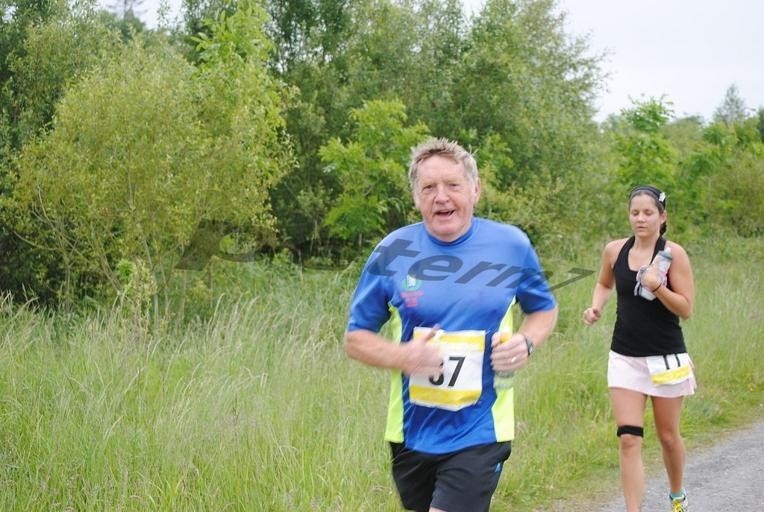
[[638, 246, 672, 301], [492, 327, 516, 378]]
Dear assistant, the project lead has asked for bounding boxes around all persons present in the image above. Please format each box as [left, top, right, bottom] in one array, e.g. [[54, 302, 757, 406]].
[[582, 186, 696, 512], [343, 138, 558, 511]]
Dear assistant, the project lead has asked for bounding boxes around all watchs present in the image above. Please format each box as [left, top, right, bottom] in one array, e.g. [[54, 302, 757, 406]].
[[524, 335, 534, 356]]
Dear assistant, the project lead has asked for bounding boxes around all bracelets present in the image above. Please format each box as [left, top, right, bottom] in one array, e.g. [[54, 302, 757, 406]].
[[652, 284, 662, 293]]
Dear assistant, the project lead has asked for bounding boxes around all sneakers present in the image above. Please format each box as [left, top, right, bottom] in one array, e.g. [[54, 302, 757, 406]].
[[669, 492, 689, 512]]
